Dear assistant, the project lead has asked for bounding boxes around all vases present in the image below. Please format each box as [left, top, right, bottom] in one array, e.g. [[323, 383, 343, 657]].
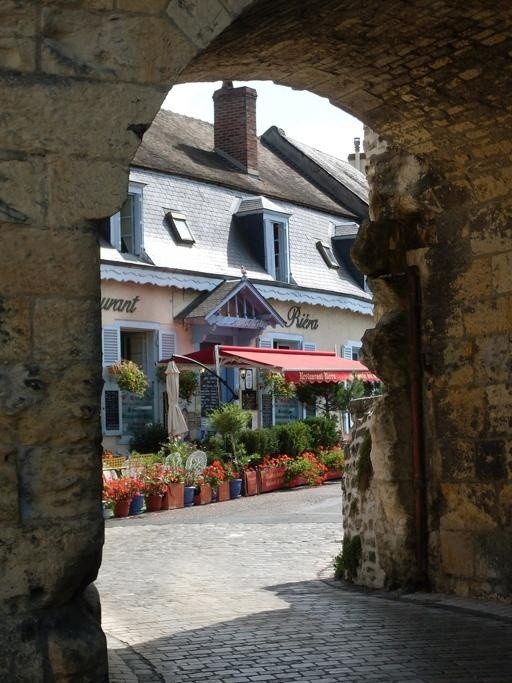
[[103, 494, 147, 518]]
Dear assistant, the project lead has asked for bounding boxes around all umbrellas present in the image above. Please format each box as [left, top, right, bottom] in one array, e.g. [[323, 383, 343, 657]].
[[164, 352, 239, 438]]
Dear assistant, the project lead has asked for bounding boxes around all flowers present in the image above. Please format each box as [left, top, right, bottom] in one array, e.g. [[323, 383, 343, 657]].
[[102, 441, 343, 509]]
